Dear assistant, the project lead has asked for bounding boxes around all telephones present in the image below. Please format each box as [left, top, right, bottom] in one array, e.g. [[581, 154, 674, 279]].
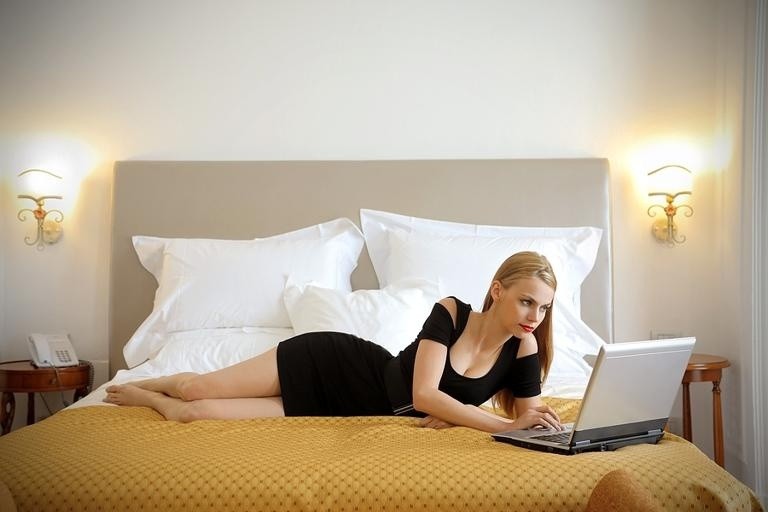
[[26, 331, 83, 369]]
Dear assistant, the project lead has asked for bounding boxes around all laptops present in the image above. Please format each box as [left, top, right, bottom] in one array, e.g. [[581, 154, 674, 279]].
[[491, 334, 698, 455]]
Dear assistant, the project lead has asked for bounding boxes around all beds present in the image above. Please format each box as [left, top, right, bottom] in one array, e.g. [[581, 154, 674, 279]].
[[1, 159, 764, 512]]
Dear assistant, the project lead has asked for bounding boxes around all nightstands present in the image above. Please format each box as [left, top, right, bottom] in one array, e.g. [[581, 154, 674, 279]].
[[682, 352, 731, 471], [0, 360, 91, 434]]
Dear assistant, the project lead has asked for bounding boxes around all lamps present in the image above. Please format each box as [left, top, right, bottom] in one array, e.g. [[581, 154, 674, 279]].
[[646, 165, 693, 245], [18, 169, 68, 251]]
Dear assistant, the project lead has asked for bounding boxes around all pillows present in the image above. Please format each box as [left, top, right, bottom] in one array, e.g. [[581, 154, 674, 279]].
[[540, 294, 608, 398], [358, 208, 604, 321], [276, 273, 456, 357], [120, 217, 365, 368], [131, 326, 294, 377]]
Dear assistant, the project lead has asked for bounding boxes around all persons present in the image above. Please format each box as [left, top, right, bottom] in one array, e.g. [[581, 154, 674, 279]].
[[102, 250, 568, 433]]
